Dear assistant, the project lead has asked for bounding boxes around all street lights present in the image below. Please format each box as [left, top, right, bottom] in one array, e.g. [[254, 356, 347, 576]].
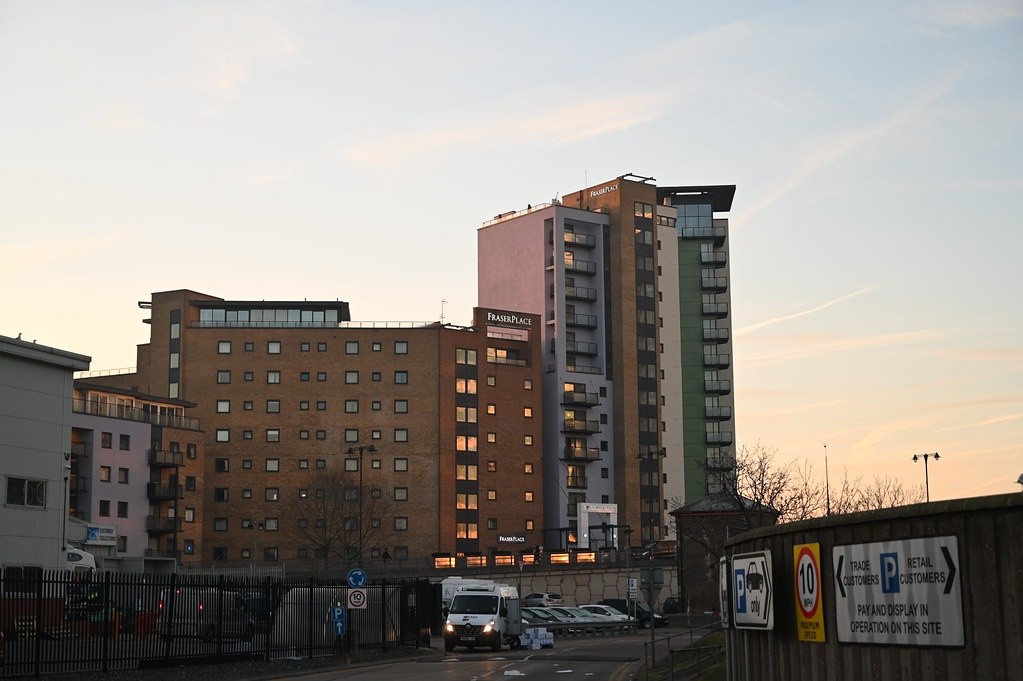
[[345, 443, 378, 569], [635, 448, 666, 537], [911, 451, 941, 500]]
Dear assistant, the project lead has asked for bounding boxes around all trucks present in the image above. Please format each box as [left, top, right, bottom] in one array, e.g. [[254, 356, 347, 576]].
[[444, 584, 522, 652], [66, 543, 100, 610]]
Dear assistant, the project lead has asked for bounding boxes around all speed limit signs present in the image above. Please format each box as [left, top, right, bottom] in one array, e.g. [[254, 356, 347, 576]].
[[795, 549, 820, 617], [350, 590, 365, 606]]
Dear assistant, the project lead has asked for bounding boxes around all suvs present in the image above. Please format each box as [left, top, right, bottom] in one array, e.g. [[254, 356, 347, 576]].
[[596, 598, 669, 629]]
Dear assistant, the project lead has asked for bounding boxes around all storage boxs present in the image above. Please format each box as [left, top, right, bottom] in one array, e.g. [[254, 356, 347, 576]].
[[520, 628, 553, 650]]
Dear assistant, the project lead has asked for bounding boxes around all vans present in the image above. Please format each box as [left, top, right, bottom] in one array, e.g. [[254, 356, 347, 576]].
[[155, 588, 256, 643], [628, 540, 677, 561]]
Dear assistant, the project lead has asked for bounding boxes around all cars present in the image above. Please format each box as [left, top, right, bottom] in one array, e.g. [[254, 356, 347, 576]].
[[521, 606, 605, 634], [522, 592, 564, 608], [578, 604, 636, 630], [663, 596, 681, 613]]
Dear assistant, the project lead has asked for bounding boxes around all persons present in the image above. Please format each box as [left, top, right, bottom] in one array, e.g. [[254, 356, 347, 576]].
[[533, 546, 541, 564], [382, 548, 392, 566]]
